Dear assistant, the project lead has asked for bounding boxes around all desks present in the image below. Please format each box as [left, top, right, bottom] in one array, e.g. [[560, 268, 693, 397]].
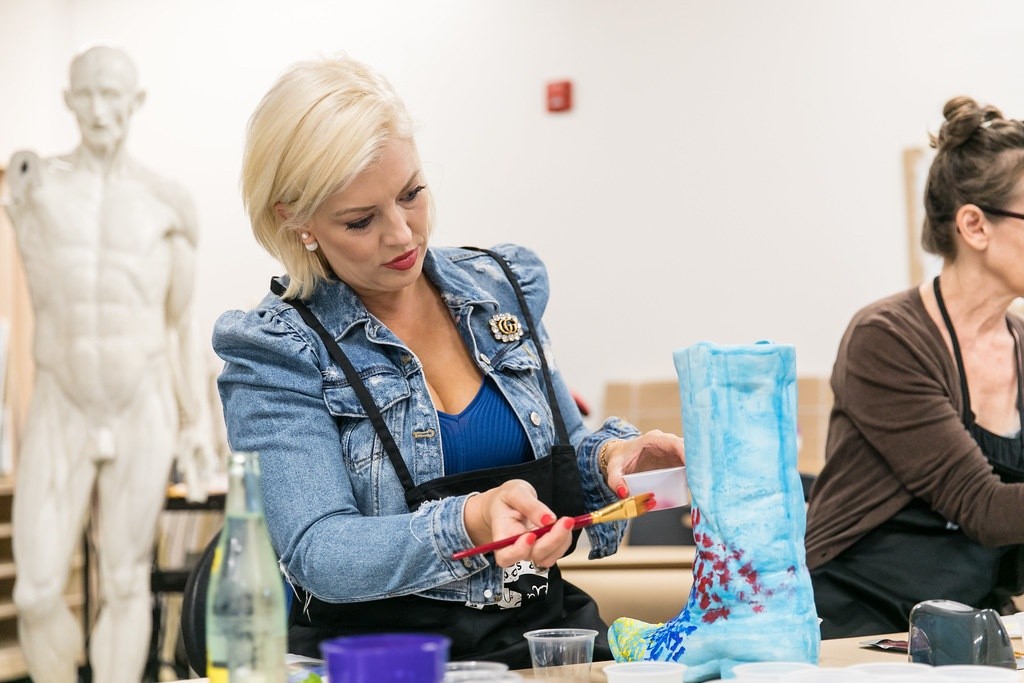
[[510, 612, 1024, 683]]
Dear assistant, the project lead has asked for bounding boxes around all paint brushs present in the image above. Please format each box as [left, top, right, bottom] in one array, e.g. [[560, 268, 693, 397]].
[[451, 492, 657, 560]]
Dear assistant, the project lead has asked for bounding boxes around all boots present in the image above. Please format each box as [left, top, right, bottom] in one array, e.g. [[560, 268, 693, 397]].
[[607, 340, 822, 683]]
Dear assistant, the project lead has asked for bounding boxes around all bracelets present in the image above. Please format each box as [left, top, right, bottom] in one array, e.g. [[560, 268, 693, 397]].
[[600, 439, 630, 471]]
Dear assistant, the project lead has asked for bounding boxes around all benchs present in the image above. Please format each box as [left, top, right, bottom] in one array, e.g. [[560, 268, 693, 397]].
[[556, 375, 832, 626]]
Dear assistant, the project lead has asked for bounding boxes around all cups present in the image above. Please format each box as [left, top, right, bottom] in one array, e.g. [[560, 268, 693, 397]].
[[621, 466, 688, 512], [318, 633, 451, 683], [603, 662, 687, 683], [712, 662, 1024, 683], [523, 628, 599, 683]]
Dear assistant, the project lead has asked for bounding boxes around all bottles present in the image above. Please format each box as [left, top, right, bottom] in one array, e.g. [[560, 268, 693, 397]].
[[207, 452, 289, 683]]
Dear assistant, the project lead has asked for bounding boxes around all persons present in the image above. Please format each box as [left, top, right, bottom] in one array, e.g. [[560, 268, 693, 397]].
[[0, 44, 221, 683], [797, 94, 1024, 645], [218, 52, 697, 663]]
